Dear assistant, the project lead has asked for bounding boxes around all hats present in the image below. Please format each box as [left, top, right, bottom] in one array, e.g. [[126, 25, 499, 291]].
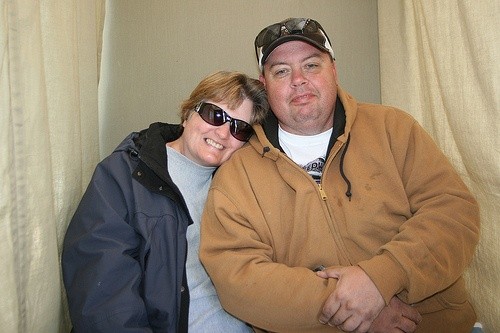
[[253, 17, 336, 73]]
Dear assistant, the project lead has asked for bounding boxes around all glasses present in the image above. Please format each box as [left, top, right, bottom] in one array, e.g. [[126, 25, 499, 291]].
[[255, 18, 333, 62], [191, 102, 255, 143]]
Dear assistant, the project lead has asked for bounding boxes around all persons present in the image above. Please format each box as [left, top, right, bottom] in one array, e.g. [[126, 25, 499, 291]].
[[199, 18, 485, 333], [61, 68, 271, 333]]
